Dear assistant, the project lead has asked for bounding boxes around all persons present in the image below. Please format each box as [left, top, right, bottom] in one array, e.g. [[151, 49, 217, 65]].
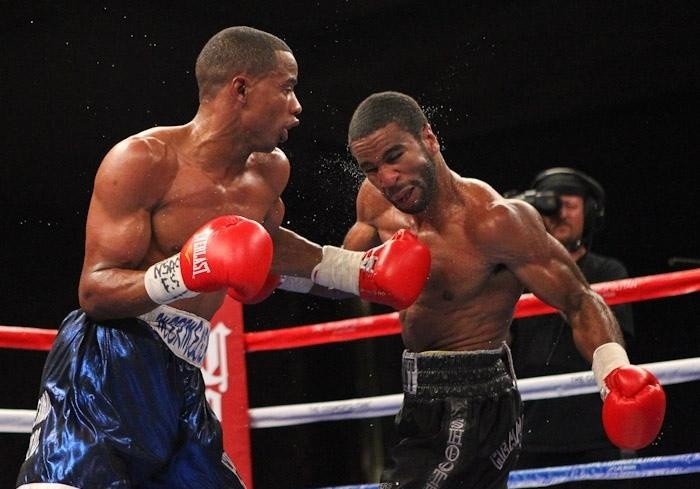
[[243, 90, 666, 489], [505, 167, 636, 471], [15, 25, 431, 489]]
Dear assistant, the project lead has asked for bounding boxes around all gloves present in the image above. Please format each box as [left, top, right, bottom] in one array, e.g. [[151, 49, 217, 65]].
[[313, 229, 431, 310], [144, 216, 274, 306], [592, 340, 666, 449]]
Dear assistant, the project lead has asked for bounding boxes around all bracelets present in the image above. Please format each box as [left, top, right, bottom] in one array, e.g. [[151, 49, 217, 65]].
[[310, 244, 366, 297], [143, 251, 199, 307], [591, 342, 630, 394]]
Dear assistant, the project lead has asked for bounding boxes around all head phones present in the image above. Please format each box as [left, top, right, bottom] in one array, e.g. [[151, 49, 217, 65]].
[[531, 167, 604, 236]]
